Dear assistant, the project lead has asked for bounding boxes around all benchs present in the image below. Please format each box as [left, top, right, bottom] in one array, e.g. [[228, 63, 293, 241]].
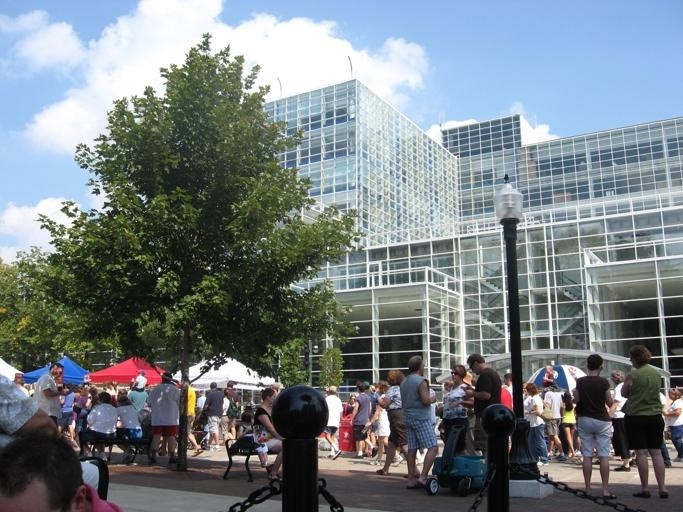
[[220, 436, 278, 482], [87, 436, 146, 466]]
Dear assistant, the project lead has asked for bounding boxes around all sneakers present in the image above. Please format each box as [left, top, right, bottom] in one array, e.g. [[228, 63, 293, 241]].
[[257, 445, 424, 481], [631, 490, 651, 498], [658, 491, 669, 498], [536, 448, 683, 472], [83, 441, 235, 467]]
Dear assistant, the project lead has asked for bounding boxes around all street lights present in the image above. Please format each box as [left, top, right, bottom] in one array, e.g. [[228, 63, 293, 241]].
[[493, 173, 540, 480]]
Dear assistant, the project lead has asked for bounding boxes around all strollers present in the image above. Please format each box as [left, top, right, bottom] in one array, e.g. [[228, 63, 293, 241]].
[[426, 416, 486, 498], [185, 410, 209, 451]]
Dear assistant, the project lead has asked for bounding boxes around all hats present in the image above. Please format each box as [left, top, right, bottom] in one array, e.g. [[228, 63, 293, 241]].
[[135, 375, 148, 388], [363, 381, 376, 394], [462, 372, 473, 386], [159, 371, 172, 381]]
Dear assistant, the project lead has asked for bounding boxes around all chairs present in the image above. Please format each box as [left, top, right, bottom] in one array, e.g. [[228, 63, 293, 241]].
[[78, 454, 109, 501]]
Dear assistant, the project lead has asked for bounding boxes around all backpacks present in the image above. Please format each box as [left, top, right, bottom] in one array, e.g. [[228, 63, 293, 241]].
[[226, 395, 238, 419]]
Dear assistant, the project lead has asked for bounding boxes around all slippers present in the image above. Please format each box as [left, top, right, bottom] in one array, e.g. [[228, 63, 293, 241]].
[[405, 478, 428, 490], [603, 491, 617, 500]]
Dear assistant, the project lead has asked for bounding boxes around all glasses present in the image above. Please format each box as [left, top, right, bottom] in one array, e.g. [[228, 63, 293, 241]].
[[450, 371, 459, 374]]
